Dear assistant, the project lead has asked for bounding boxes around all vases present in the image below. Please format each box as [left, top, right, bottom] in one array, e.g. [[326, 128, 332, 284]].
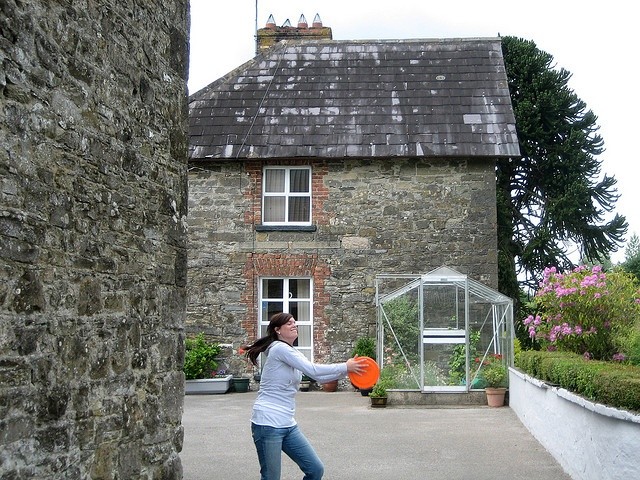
[[231, 377, 249, 392], [322, 377, 338, 392]]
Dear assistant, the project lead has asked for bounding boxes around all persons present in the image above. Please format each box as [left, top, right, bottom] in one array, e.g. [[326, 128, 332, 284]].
[[241, 312, 370, 480]]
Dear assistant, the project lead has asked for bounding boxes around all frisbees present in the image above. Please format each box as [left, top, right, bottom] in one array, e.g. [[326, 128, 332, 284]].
[[350, 357, 380, 390]]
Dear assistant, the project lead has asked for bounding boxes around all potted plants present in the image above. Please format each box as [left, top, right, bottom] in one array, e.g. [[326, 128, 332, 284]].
[[369, 383, 388, 408], [472, 354, 506, 407], [299, 376, 311, 392], [184, 331, 232, 394], [352, 336, 376, 396]]
